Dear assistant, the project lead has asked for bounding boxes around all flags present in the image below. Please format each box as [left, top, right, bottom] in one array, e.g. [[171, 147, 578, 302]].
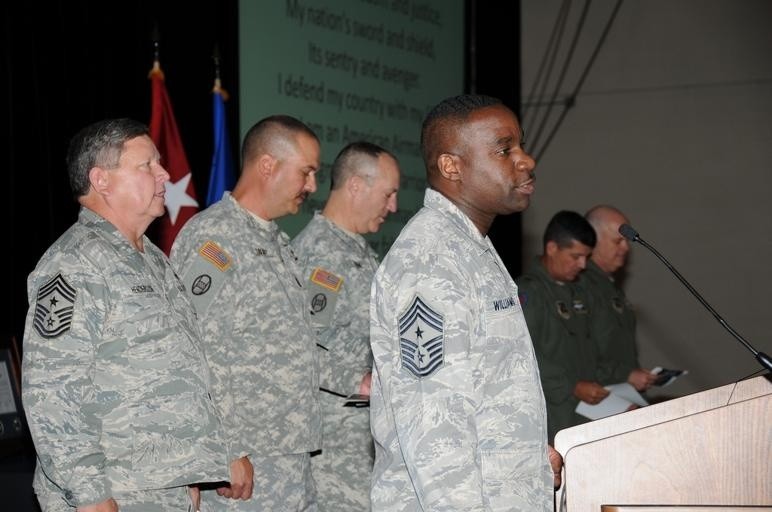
[[140, 44, 202, 260], [205, 57, 243, 211]]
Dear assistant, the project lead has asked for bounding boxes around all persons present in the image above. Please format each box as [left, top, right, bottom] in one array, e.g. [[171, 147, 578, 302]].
[[513, 208, 643, 446], [289, 139, 402, 511], [166, 113, 329, 511], [566, 204, 670, 414], [18, 114, 234, 512], [360, 90, 565, 511]]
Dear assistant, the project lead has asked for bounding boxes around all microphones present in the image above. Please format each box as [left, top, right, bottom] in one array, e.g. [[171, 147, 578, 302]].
[[617, 221, 772, 375]]
